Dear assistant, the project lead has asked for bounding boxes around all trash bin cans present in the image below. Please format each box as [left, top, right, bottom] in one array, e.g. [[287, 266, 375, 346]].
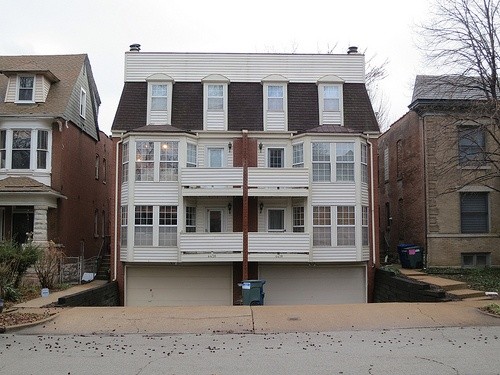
[[403, 245, 423, 269], [241, 279, 266, 306], [396, 243, 417, 268]]
